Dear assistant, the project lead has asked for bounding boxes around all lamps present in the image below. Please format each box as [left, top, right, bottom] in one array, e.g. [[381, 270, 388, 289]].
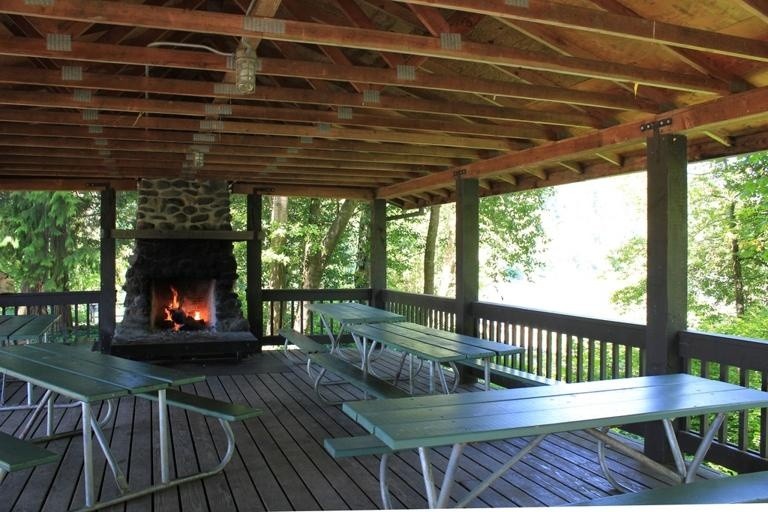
[[234, 35, 257, 96]]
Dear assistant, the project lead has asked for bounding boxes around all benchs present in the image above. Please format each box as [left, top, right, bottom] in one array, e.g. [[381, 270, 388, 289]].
[[324, 427, 628, 509], [276, 302, 567, 407], [544, 465, 766, 507], [0, 429, 56, 484], [137, 384, 262, 472]]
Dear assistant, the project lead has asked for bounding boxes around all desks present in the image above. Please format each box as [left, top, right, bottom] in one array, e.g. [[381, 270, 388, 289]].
[[0, 312, 59, 411], [338, 368, 768, 510], [1, 343, 212, 508]]
